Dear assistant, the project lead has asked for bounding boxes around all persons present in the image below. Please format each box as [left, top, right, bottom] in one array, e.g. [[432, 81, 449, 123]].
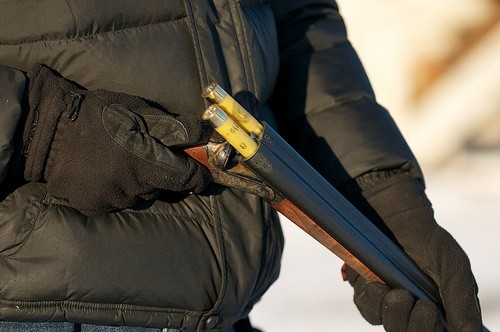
[[1, 1, 482, 330]]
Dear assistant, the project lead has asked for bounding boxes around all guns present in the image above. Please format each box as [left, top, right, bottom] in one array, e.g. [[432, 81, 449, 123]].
[[182, 83, 488, 331]]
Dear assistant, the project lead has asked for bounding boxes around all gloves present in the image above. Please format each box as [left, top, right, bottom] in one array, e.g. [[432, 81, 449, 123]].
[[347, 170, 485, 332], [14, 63, 215, 218]]
